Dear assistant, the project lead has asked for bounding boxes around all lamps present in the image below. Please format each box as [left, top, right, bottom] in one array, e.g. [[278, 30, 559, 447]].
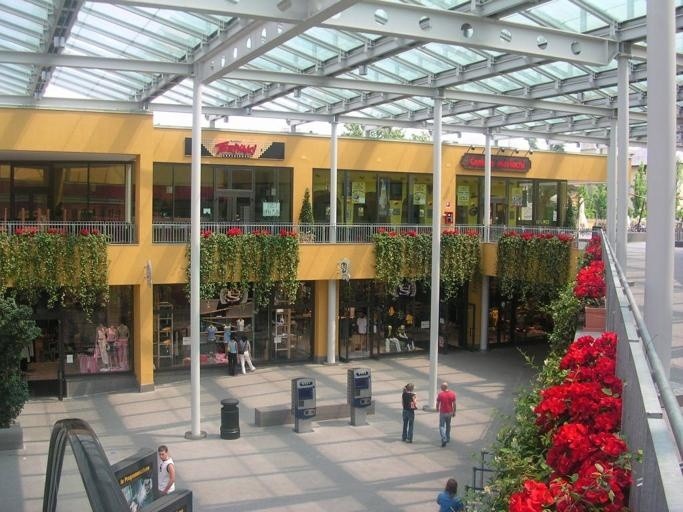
[[466, 144, 532, 157]]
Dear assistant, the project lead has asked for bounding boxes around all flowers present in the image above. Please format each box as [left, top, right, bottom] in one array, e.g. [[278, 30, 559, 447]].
[[485, 331, 633, 512], [547, 235, 608, 340]]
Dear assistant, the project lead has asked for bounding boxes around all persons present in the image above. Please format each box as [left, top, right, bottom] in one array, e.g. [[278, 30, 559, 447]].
[[237, 336, 256, 374], [436, 479, 462, 512], [382, 324, 415, 353], [354, 311, 367, 351], [226, 337, 238, 375], [435, 381, 456, 447], [95, 322, 131, 371], [157, 446, 175, 495], [205, 315, 244, 343], [401, 383, 417, 443]]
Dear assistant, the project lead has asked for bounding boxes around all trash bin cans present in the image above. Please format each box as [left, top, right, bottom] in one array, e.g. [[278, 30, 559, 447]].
[[221, 399, 239, 439]]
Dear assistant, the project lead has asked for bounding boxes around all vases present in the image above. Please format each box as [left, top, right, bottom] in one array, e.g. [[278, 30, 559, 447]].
[[582, 304, 608, 331]]
